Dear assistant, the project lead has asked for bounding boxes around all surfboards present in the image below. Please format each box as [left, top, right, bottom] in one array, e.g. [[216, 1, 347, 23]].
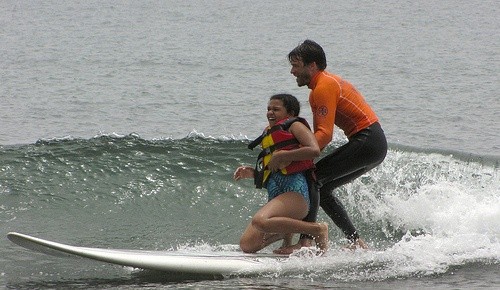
[[7, 233, 400, 276]]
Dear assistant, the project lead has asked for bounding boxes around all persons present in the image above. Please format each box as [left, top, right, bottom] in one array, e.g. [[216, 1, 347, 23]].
[[232, 94, 329, 256], [278, 39, 388, 254]]
